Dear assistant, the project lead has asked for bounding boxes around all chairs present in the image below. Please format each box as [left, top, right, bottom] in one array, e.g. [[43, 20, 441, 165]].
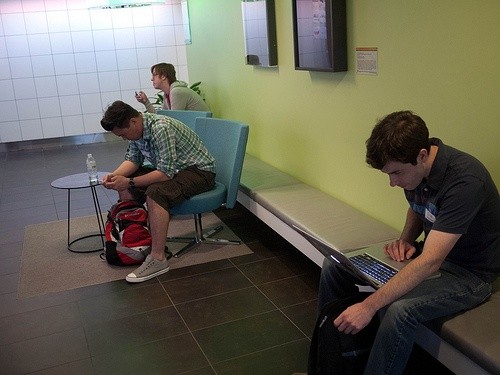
[[156, 109, 212, 130], [161, 116, 248, 260]]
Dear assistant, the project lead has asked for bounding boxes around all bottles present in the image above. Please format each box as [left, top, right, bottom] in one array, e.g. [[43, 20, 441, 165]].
[[86, 154, 99, 183]]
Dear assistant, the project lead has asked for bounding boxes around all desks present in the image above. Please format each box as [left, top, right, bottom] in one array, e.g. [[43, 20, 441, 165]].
[[51, 172, 117, 253]]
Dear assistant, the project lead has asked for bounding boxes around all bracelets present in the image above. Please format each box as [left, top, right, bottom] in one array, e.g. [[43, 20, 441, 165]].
[[128, 178, 134, 190]]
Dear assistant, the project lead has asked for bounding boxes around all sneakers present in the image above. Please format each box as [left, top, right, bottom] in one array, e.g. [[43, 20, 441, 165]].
[[125, 254, 170, 282]]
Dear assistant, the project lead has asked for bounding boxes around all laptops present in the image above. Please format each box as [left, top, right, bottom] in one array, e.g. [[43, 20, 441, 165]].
[[293, 225, 442, 289]]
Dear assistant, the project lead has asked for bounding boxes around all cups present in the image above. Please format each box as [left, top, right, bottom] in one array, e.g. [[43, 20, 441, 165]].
[[248, 55, 259, 65]]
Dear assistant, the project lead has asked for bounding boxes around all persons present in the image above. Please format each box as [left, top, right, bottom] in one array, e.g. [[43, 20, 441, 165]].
[[135, 63, 208, 113], [316, 110, 500, 375], [100, 100, 217, 282]]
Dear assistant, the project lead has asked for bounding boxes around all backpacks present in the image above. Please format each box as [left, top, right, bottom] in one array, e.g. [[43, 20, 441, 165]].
[[306, 297, 383, 375], [102, 199, 173, 267]]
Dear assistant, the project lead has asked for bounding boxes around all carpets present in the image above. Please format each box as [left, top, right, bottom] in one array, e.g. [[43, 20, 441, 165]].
[[20, 207, 254, 298]]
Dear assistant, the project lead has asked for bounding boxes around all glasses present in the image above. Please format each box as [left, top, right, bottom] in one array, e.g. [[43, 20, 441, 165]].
[[152, 72, 160, 77]]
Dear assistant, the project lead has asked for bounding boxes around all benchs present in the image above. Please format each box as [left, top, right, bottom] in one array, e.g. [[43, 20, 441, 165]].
[[237, 153, 500, 375]]
